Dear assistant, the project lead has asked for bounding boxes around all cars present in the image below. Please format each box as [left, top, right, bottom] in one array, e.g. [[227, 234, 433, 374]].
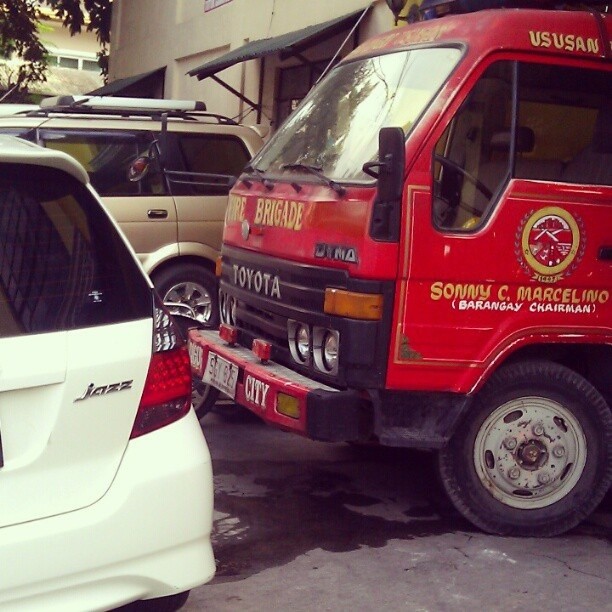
[[0, 131, 219, 611]]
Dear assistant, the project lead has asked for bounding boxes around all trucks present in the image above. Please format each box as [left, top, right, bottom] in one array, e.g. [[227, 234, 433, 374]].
[[185, 1, 611, 541]]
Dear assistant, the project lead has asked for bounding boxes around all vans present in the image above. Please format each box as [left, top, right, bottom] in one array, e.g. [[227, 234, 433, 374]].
[[0, 92, 277, 332]]
